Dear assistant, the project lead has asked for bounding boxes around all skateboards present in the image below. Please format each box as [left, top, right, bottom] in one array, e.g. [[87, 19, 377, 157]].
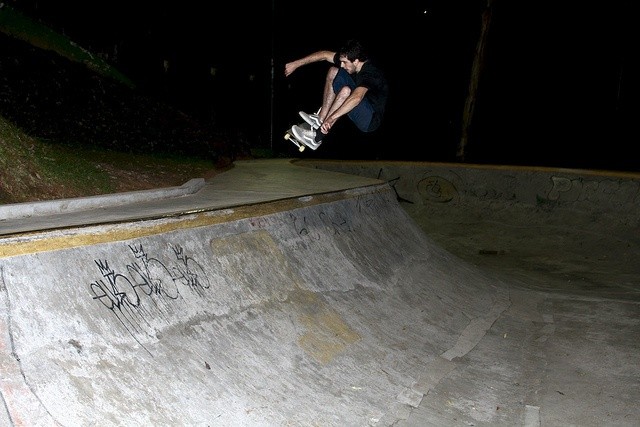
[[285, 122, 322, 153]]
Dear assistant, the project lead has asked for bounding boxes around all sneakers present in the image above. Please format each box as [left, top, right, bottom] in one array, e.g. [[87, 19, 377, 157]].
[[299, 108, 325, 129], [291, 125, 322, 150]]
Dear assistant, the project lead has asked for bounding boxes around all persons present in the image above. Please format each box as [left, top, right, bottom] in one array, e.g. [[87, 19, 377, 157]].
[[285, 40, 388, 150]]
